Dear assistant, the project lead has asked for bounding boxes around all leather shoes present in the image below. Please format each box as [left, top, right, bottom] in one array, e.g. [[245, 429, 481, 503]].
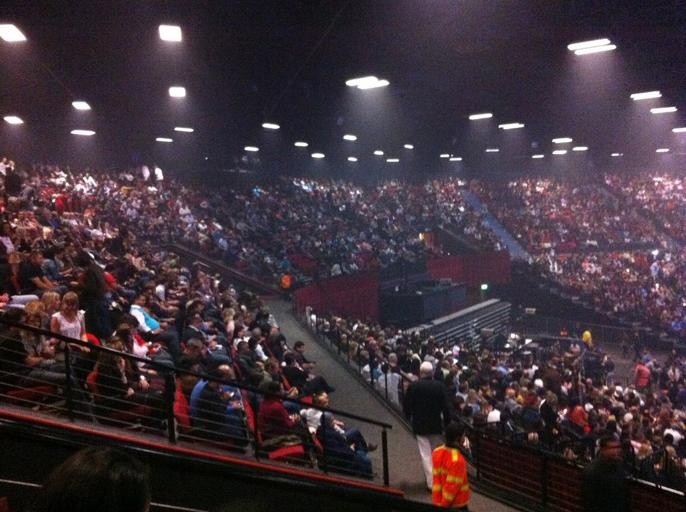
[[367, 442, 377, 452]]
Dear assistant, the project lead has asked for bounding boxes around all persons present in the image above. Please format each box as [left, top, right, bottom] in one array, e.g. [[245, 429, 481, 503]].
[[1, 157, 685, 511]]
